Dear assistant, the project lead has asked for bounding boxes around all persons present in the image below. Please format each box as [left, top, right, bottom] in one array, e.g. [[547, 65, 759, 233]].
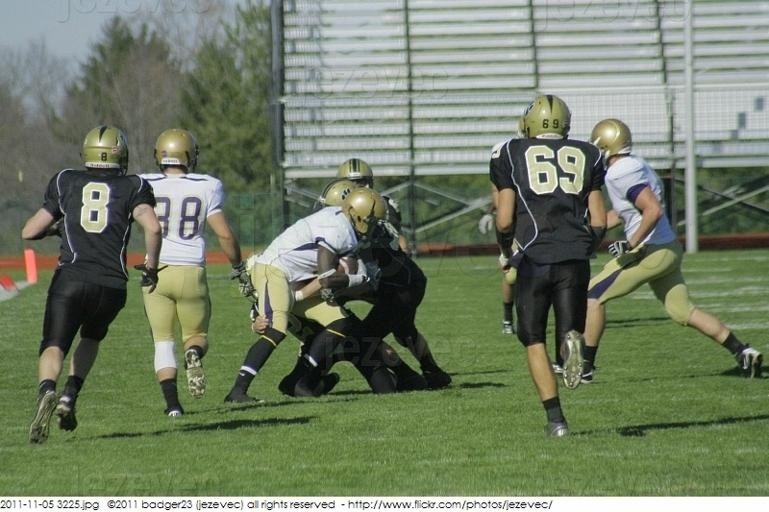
[[138, 129, 252, 416], [22, 124, 162, 444], [551, 118, 762, 384], [221, 159, 446, 404], [496, 97, 607, 437], [477, 199, 520, 334]]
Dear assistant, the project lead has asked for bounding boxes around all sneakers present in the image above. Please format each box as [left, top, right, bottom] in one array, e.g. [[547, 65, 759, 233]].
[[543, 422, 568, 437], [184, 349, 206, 399], [367, 365, 451, 394], [733, 343, 762, 378], [55, 395, 77, 431], [320, 259, 367, 303], [223, 389, 263, 404], [552, 330, 593, 389], [277, 369, 339, 402], [29, 390, 58, 444], [164, 405, 183, 417], [502, 321, 513, 334]]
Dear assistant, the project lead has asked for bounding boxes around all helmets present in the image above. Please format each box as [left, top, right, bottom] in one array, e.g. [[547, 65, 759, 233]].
[[154, 129, 199, 172], [239, 254, 263, 302], [83, 125, 128, 175], [318, 159, 386, 242], [518, 95, 570, 138], [590, 119, 631, 159]]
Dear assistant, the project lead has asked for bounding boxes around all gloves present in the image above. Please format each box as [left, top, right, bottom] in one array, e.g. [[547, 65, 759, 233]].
[[231, 261, 250, 295], [134, 261, 158, 293], [608, 241, 632, 257]]
